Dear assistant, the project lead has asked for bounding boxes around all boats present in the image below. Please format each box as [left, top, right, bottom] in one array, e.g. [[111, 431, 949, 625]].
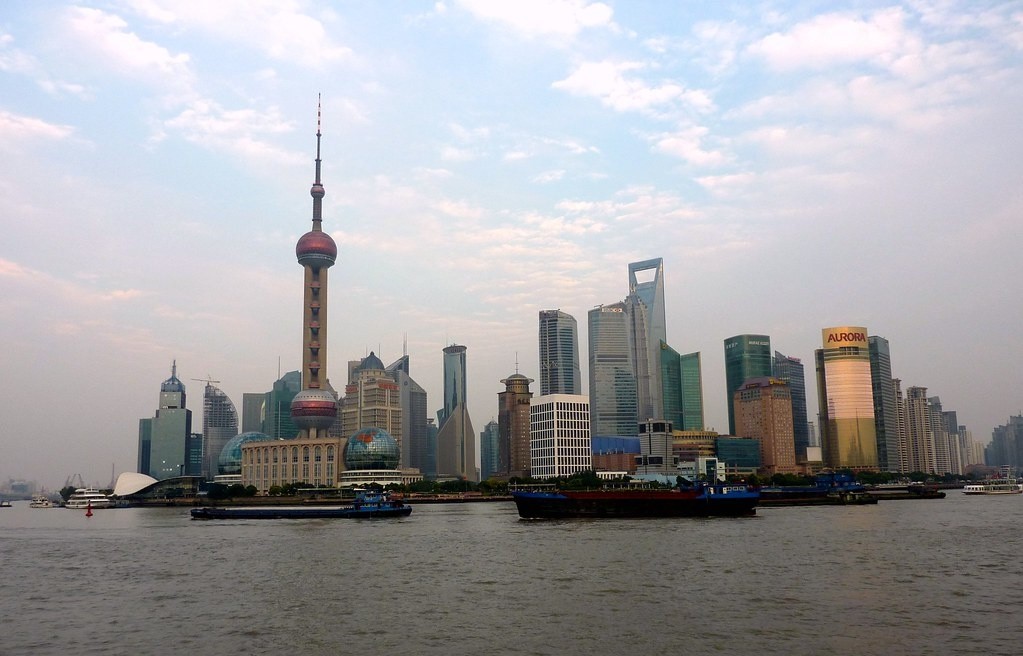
[[0, 500, 12, 507], [962, 479, 1023, 495], [65, 486, 116, 509], [191, 500, 412, 520], [29, 495, 53, 508], [758, 474, 864, 505], [511, 482, 761, 518], [864, 483, 947, 500]]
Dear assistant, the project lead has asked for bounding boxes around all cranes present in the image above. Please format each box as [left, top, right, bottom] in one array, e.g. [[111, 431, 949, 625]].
[[191, 378, 220, 385]]
